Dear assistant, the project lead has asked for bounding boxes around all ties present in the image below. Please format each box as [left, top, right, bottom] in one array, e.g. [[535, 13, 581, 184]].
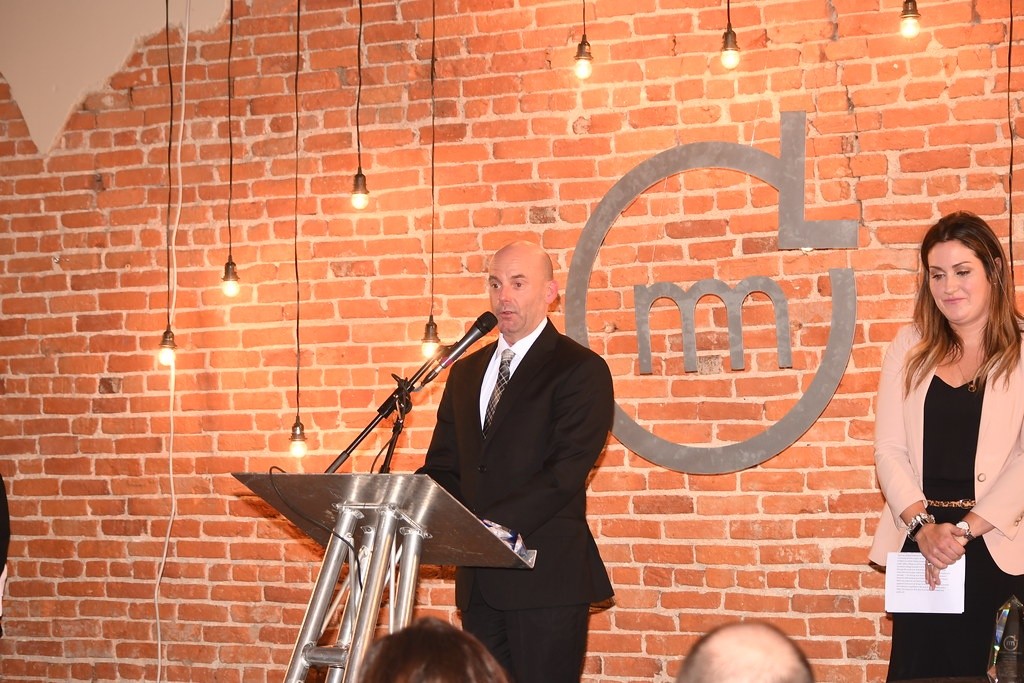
[[483, 348, 516, 440]]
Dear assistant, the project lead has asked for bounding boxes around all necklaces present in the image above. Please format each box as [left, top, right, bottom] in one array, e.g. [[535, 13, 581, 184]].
[[957, 362, 983, 392]]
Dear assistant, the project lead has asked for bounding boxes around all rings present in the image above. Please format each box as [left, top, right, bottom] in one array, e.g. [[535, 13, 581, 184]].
[[926, 560, 933, 566]]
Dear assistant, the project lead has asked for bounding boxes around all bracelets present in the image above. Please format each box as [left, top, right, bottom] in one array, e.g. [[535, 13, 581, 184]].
[[905, 512, 935, 544]]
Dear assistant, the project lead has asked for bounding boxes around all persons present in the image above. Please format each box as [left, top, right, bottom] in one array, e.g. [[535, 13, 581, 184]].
[[676, 619, 814, 682], [358, 616, 510, 683], [866, 210, 1024, 683], [0, 473, 10, 638], [414, 242, 615, 683]]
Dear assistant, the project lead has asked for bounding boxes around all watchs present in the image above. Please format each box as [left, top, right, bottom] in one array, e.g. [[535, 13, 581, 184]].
[[955, 521, 976, 540]]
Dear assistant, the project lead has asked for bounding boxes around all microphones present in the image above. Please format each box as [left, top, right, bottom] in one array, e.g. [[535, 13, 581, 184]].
[[421, 311, 498, 385]]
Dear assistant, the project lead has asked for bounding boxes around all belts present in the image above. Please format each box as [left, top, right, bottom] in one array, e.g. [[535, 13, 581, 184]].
[[927, 499, 975, 509]]
